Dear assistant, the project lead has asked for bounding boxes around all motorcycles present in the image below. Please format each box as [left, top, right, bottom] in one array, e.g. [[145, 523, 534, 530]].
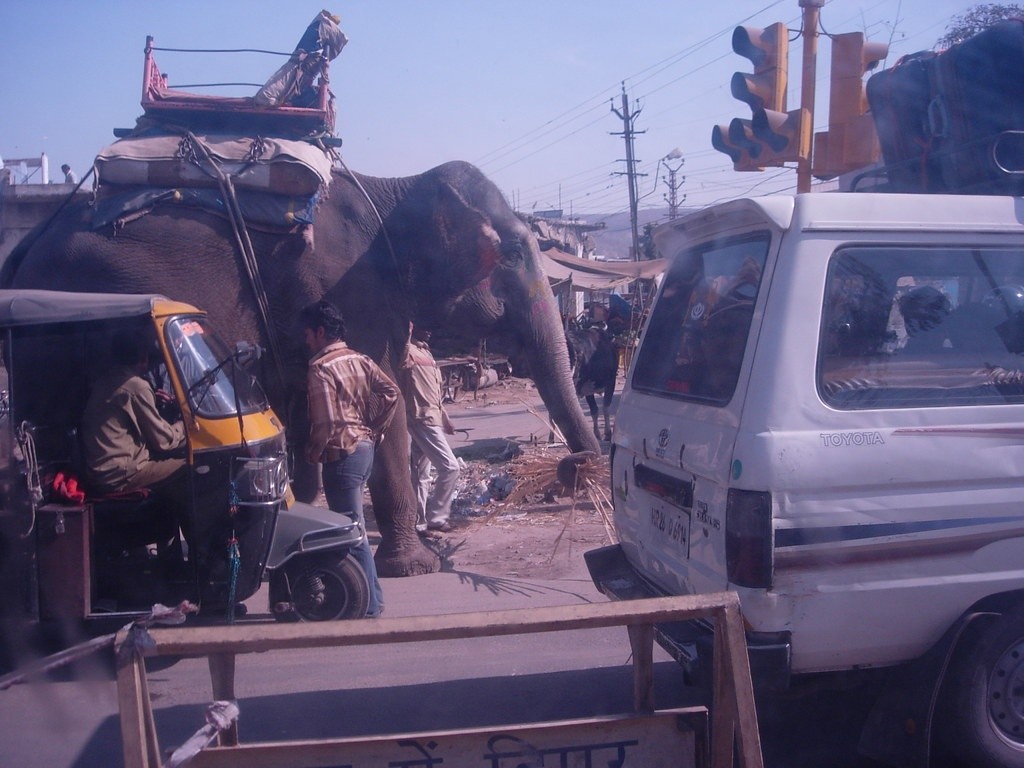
[[1, 288, 369, 641]]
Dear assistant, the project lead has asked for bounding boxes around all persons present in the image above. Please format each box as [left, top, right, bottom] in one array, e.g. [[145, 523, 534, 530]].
[[60, 163, 79, 185], [398, 312, 458, 541], [80, 326, 246, 618], [295, 300, 402, 615], [893, 286, 951, 363]]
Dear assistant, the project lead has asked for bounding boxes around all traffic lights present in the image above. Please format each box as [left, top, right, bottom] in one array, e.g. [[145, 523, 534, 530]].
[[711, 107, 810, 171], [812, 31, 888, 180], [730, 22, 788, 115]]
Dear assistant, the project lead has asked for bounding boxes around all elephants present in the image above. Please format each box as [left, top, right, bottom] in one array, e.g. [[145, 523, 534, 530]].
[[0, 157, 604, 580]]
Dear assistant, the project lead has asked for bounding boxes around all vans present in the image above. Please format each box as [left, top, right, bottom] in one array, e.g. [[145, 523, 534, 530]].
[[583, 192, 1024, 768]]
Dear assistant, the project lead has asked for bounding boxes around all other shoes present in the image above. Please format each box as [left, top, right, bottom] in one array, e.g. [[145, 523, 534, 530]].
[[416, 531, 445, 543], [427, 523, 458, 533]]
